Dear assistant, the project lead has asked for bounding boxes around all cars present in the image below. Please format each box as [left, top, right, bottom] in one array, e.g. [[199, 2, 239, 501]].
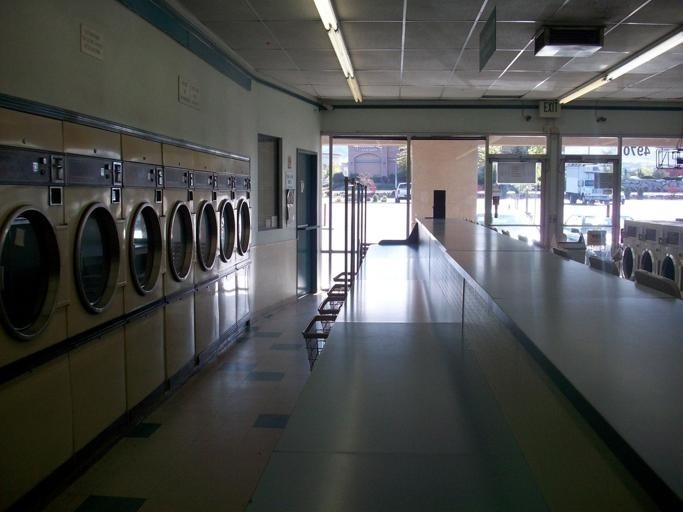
[[394, 182, 411, 202]]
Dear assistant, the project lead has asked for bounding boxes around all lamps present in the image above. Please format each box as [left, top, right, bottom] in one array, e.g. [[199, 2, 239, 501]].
[[312, 0, 363, 104], [556, 27, 683, 105]]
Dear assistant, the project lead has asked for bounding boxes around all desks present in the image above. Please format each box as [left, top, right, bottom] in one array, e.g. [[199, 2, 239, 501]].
[[243, 215, 683, 511]]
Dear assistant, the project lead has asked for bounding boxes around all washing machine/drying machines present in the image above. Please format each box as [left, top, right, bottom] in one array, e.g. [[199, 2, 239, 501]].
[[621, 219, 683, 287], [1, 98, 252, 511]]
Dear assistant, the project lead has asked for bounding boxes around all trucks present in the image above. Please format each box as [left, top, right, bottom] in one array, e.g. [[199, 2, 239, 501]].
[[565, 163, 626, 204]]
